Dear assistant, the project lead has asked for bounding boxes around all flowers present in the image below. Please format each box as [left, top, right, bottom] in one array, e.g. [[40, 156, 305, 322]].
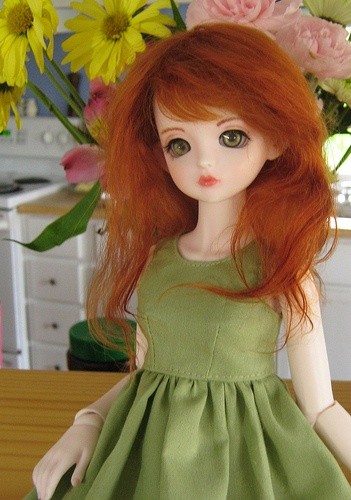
[[0, 1, 351, 173]]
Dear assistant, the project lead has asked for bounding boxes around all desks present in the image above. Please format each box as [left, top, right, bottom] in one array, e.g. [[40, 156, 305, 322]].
[[0, 367, 351, 499]]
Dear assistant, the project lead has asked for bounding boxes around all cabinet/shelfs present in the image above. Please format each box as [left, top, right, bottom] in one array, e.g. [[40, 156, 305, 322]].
[[17, 183, 351, 382]]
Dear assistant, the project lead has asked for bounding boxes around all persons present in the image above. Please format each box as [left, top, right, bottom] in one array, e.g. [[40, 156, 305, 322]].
[[17, 23, 350, 500]]
